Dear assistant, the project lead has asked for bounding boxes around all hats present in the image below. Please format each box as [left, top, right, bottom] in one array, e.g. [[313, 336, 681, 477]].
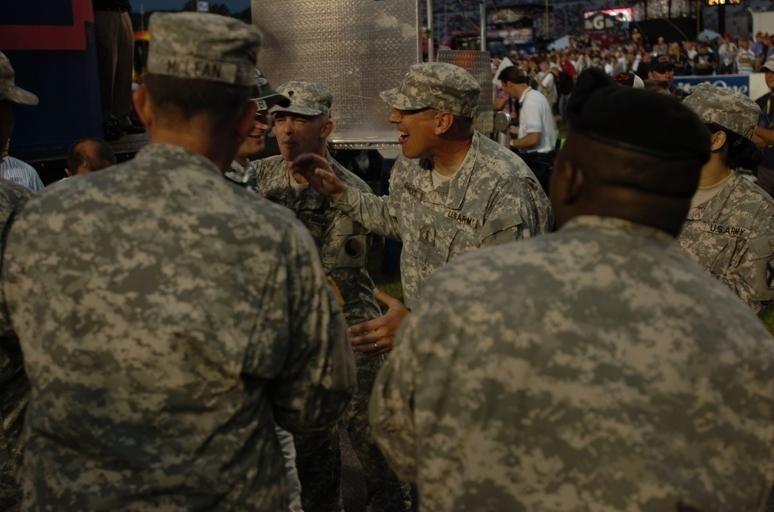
[[145, 9, 264, 88], [565, 54, 714, 164], [267, 80, 334, 117], [758, 54, 774, 73], [1, 52, 40, 108], [682, 81, 761, 141], [378, 61, 482, 120]]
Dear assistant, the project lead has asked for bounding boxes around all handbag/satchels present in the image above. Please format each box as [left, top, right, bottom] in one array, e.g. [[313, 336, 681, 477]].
[[543, 88, 557, 108]]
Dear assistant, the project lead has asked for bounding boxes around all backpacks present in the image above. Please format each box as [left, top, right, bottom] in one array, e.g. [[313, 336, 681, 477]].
[[559, 72, 574, 96]]
